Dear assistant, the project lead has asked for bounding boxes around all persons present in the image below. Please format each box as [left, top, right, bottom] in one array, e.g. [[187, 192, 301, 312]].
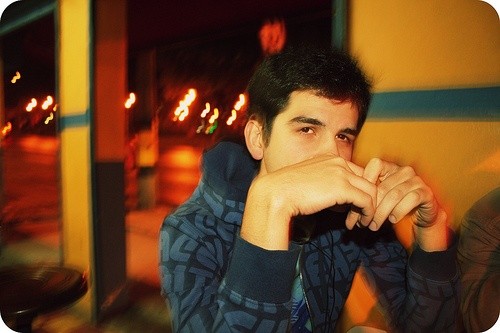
[[154, 38, 465, 333]]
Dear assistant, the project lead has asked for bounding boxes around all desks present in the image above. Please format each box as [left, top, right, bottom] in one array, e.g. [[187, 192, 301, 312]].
[[1, 264, 89, 332]]
